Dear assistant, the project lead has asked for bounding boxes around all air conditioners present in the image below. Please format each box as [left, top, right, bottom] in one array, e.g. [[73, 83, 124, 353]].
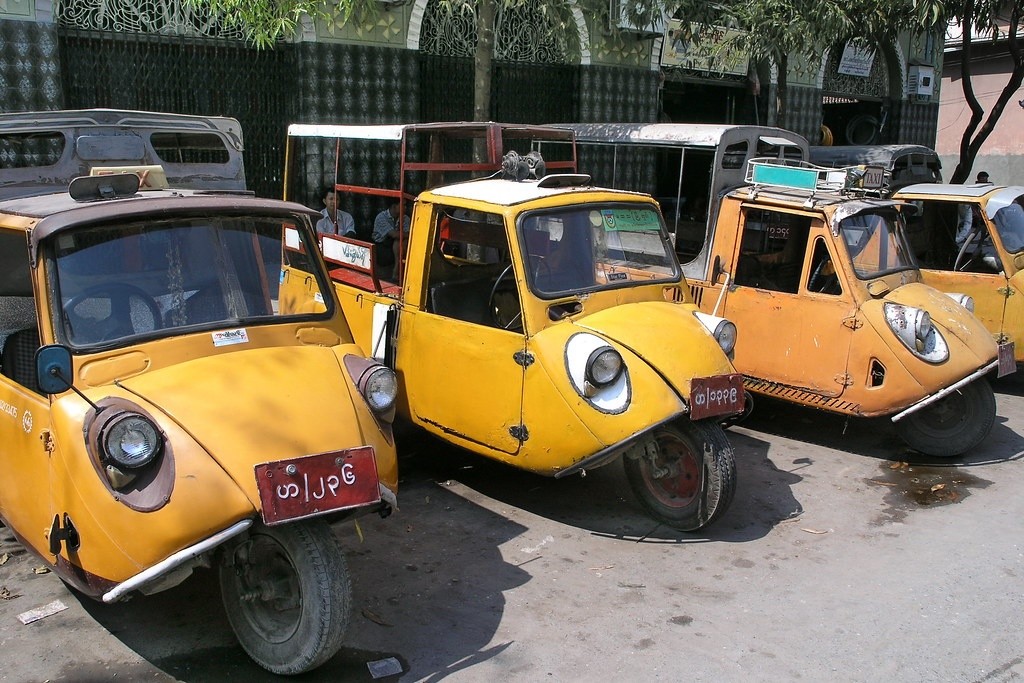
[[609, 0, 665, 36]]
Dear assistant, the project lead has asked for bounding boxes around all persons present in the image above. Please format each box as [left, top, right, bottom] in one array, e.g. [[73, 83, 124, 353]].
[[955, 171, 1006, 245], [372, 196, 410, 284], [316, 189, 357, 239], [440, 214, 450, 253]]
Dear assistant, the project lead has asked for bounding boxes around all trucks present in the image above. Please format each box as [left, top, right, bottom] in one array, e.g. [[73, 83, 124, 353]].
[[805, 141, 1024, 379], [524, 120, 1018, 461], [271, 117, 755, 535], [0, 107, 403, 678]]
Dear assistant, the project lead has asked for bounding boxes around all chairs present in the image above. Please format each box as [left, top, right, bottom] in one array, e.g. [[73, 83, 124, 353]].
[[0, 327, 48, 400], [430, 276, 496, 325]]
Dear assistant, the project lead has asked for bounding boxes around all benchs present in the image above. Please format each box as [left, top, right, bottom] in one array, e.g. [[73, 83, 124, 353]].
[[283, 223, 402, 301]]
